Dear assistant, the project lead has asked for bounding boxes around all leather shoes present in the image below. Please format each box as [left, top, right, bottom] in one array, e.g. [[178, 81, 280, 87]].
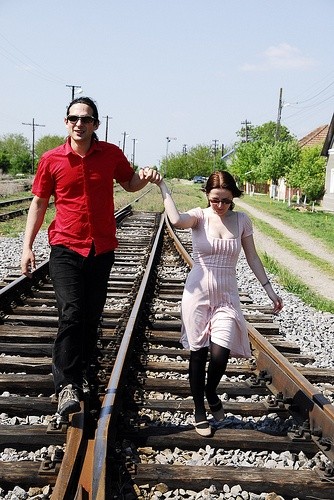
[[208, 402, 227, 424], [192, 418, 212, 437]]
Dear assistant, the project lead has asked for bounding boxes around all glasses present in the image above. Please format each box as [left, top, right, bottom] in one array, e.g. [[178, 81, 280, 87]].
[[209, 199, 234, 205], [66, 114, 96, 123]]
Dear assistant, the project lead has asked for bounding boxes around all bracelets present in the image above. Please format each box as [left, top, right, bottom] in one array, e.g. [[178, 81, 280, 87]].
[[262, 280, 269, 287]]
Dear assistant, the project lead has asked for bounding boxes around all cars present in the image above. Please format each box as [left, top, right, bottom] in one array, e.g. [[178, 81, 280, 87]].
[[194, 176, 204, 183]]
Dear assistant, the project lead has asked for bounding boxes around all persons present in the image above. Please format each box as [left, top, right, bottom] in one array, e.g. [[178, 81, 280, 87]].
[[19, 97, 163, 417], [139, 167, 284, 438]]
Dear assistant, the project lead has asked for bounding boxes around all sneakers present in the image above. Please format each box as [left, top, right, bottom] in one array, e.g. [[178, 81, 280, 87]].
[[55, 384, 80, 417], [79, 380, 93, 399]]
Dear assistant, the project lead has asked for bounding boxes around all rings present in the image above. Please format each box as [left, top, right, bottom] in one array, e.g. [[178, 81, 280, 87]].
[[156, 171, 161, 176], [140, 167, 145, 171]]
